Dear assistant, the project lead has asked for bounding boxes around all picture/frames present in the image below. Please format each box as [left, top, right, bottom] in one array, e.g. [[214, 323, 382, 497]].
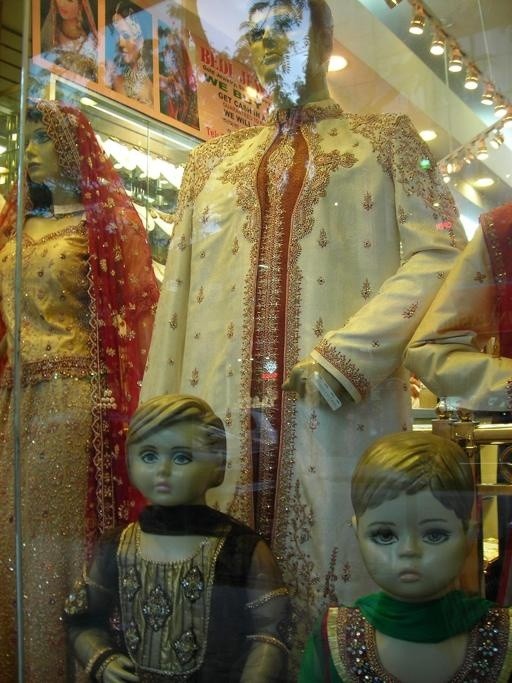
[[28, 1, 208, 143]]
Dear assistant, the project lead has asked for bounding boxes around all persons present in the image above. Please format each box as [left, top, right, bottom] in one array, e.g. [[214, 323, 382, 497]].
[[401, 206, 512, 414], [41, 1, 98, 84], [61, 392, 291, 683], [299, 432, 512, 683], [137, 1, 469, 683], [1, 101, 163, 683], [111, 2, 154, 107]]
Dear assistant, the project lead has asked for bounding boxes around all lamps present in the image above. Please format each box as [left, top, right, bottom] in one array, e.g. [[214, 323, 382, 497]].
[[384, 1, 512, 184]]
